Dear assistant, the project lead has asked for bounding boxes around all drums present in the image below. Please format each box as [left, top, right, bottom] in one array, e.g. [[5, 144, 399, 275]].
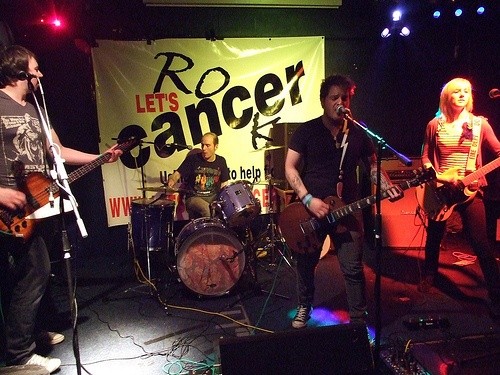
[[174, 216, 247, 296], [216, 180, 262, 229], [131, 198, 176, 252]]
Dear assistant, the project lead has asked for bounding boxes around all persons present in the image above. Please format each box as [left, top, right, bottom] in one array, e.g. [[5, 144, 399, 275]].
[[166, 132, 231, 218], [0, 43, 122, 373], [285, 75, 404, 347], [417, 78, 500, 305]]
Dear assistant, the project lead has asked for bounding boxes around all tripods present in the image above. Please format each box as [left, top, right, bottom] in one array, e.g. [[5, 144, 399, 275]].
[[227, 180, 295, 308], [104, 138, 182, 316]]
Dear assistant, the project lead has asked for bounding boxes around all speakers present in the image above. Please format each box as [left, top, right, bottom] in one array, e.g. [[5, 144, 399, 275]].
[[219, 320, 376, 375], [371, 179, 427, 250]]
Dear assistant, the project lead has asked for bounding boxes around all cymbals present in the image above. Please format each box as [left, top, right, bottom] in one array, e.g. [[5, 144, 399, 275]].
[[252, 144, 286, 152], [250, 179, 288, 185], [137, 187, 195, 194]]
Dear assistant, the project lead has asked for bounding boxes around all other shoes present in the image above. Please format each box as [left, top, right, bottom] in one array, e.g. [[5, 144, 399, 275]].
[[33, 330, 65, 346], [24, 353, 61, 375]]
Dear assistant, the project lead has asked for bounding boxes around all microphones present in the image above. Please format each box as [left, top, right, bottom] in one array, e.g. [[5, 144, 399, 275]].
[[489, 88, 500, 99], [336, 104, 348, 115], [221, 255, 234, 261], [16, 71, 37, 80], [174, 143, 192, 150], [414, 206, 421, 217]]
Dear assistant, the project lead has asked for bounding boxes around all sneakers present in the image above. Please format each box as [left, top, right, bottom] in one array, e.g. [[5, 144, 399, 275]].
[[292, 305, 314, 328]]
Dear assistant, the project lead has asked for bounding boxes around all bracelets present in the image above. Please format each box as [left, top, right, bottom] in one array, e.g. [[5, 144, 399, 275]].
[[301, 193, 312, 205]]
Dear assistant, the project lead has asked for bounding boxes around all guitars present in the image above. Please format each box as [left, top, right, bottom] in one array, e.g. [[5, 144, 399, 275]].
[[414, 157, 500, 222], [277, 164, 437, 253], [0, 137, 144, 245]]
